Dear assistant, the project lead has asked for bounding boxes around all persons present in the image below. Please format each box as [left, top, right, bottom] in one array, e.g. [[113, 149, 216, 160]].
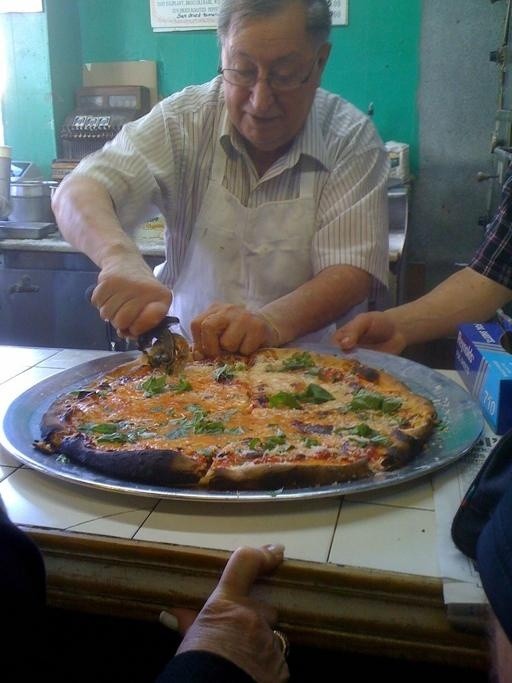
[[50, 0, 390, 358], [0, 500, 289, 682], [329, 186, 512, 645]]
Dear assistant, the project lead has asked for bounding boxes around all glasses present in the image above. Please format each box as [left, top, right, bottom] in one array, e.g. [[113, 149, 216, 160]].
[[216, 45, 323, 96]]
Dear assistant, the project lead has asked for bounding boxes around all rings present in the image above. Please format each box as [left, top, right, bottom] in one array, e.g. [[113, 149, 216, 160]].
[[273, 630, 288, 655]]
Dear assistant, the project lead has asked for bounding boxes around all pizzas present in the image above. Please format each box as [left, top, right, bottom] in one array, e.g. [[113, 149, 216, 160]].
[[39, 332, 437, 490]]
